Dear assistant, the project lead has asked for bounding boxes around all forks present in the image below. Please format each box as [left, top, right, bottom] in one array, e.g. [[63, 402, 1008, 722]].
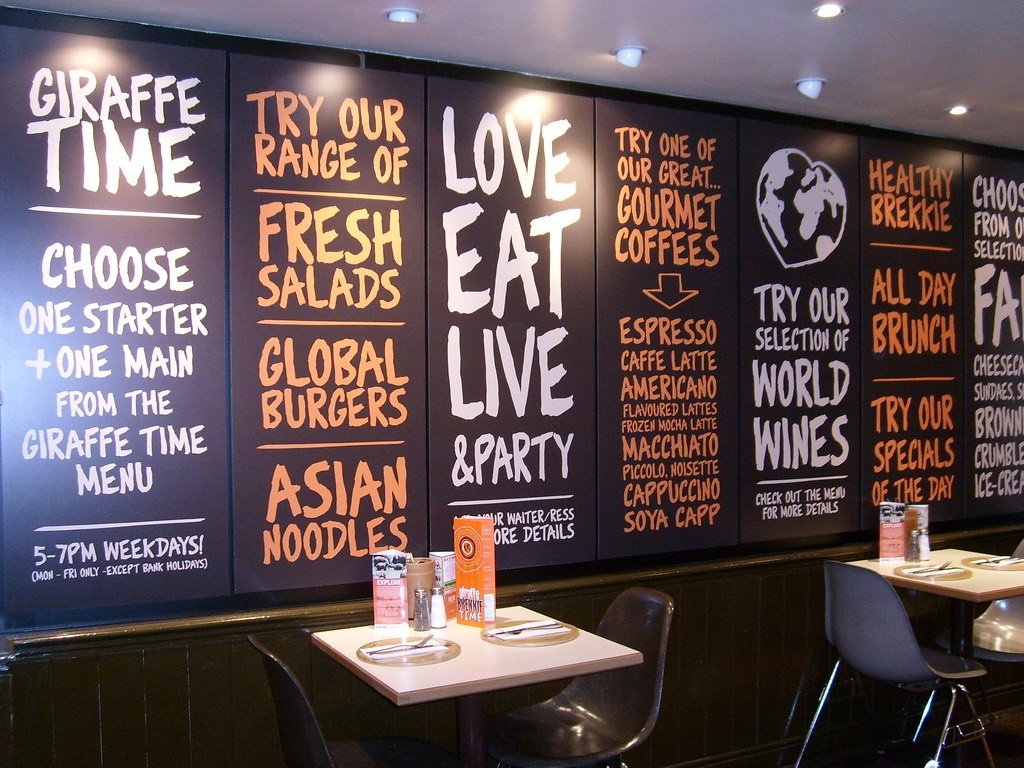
[[908, 561, 961, 574], [370, 634, 433, 655]]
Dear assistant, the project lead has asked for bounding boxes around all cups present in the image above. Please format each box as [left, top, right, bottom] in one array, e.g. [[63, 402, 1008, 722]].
[[905, 510, 918, 556], [407, 557, 434, 620]]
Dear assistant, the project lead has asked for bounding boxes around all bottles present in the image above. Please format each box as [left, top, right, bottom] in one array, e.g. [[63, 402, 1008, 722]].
[[414, 589, 429, 630], [920, 530, 930, 560], [430, 588, 446, 628], [905, 530, 920, 562]]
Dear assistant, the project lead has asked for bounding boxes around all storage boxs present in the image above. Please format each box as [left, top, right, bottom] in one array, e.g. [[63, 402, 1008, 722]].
[[454, 518, 497, 630]]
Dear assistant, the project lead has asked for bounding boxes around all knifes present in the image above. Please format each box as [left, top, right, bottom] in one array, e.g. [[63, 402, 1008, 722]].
[[485, 623, 562, 637], [367, 644, 451, 653]]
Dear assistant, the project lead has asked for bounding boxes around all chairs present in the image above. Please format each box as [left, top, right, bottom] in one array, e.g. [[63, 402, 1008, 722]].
[[244, 630, 465, 768], [777, 558, 996, 768], [931, 538, 1024, 730], [473, 587, 675, 768]]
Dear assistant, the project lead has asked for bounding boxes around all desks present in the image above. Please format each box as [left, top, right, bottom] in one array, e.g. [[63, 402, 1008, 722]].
[[309, 606, 645, 768], [841, 546, 1024, 768]]
